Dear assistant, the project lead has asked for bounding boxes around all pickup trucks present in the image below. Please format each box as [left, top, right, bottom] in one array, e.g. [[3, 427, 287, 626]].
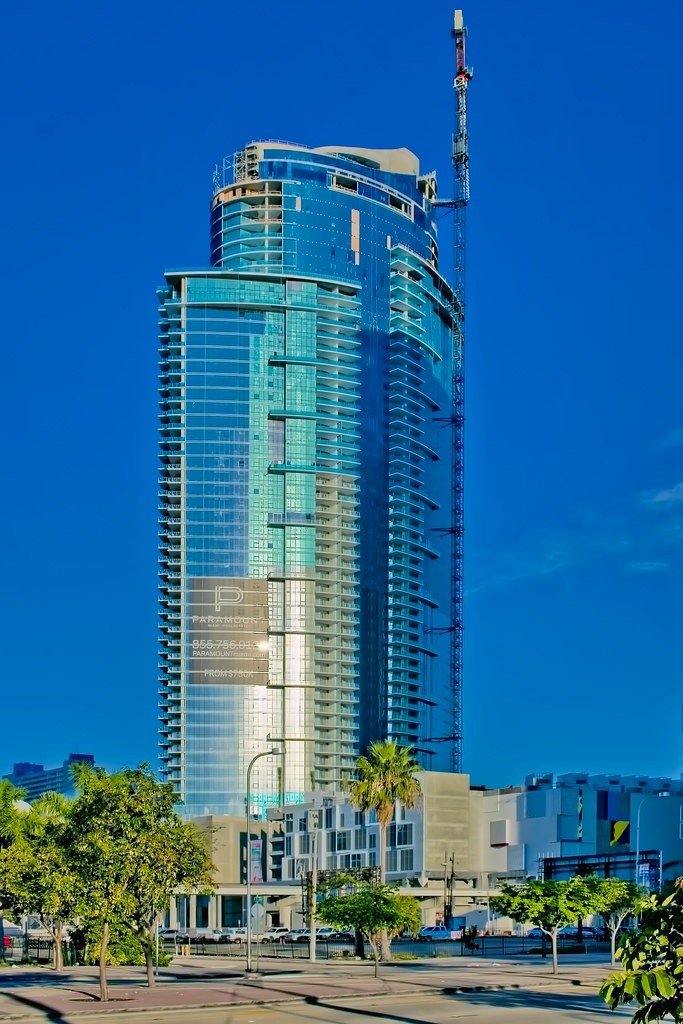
[[417, 925, 463, 943]]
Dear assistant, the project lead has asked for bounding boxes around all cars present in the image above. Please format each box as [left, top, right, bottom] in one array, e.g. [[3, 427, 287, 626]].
[[158, 926, 355, 946], [526, 925, 640, 942]]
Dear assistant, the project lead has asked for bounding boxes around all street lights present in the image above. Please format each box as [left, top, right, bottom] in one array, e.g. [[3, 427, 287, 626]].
[[634, 790, 671, 937], [246, 746, 286, 972]]
